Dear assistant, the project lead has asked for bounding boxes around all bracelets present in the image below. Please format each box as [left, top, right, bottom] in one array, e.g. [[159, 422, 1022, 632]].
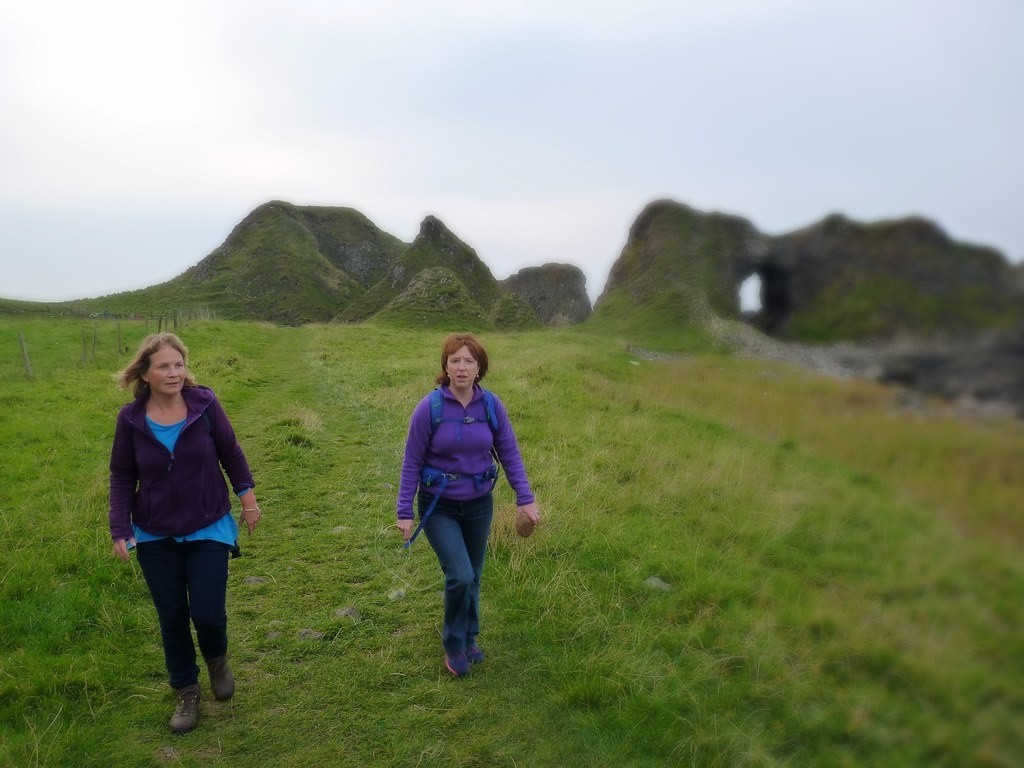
[[240, 507, 259, 512]]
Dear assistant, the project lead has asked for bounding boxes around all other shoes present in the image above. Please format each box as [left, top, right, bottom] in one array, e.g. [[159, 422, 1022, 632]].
[[204, 657, 235, 701], [168, 684, 202, 733]]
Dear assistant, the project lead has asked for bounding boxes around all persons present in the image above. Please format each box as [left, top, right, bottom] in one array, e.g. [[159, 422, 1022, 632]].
[[109, 332, 260, 734], [396, 334, 540, 676]]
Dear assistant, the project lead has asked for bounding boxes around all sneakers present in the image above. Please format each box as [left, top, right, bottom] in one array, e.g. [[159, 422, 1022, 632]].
[[466, 646, 485, 664], [444, 650, 470, 677]]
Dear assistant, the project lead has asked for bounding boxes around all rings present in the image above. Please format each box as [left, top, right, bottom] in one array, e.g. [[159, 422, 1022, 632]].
[[537, 510, 540, 515]]
